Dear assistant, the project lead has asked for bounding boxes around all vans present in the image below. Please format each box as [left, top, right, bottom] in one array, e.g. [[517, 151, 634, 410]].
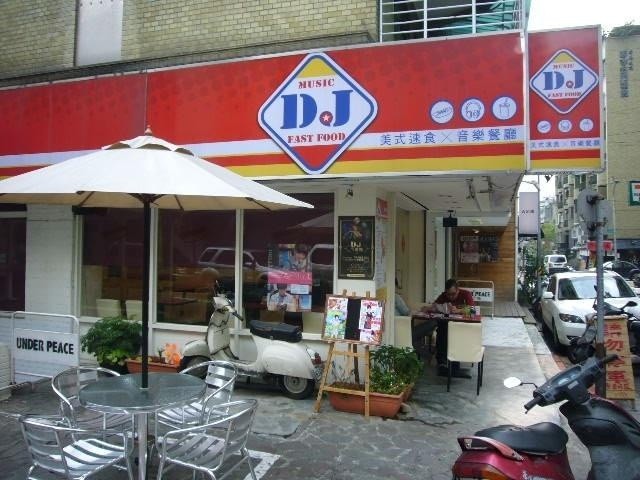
[[543, 254, 567, 274], [303, 243, 335, 297]]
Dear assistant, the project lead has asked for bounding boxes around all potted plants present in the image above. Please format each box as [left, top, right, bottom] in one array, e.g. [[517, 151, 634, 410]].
[[83, 310, 144, 372], [324, 345, 424, 419]]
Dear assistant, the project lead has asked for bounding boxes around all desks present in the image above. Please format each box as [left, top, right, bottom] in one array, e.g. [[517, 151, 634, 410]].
[[410, 304, 482, 375]]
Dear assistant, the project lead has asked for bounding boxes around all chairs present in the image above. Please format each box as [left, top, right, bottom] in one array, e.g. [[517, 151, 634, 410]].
[[97, 276, 326, 335], [17, 357, 262, 480], [443, 320, 486, 396], [393, 313, 423, 367]]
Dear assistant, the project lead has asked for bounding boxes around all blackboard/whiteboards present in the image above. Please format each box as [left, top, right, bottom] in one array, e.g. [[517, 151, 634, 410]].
[[321, 295, 385, 346], [455, 233, 499, 263]]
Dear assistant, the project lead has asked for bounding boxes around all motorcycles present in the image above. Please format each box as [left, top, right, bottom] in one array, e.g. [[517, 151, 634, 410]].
[[451, 353, 640, 480], [568, 285, 640, 377], [180, 281, 324, 399]]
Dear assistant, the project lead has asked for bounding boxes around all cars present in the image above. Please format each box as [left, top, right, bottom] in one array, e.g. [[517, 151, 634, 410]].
[[191, 247, 308, 295], [517, 269, 551, 312], [538, 270, 640, 355], [584, 260, 640, 281], [627, 268, 640, 288]]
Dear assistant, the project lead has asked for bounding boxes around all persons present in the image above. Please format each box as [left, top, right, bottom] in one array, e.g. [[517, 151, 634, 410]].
[[362, 305, 375, 334], [394, 276, 439, 341], [432, 280, 474, 367], [268, 283, 297, 313], [282, 243, 308, 271], [330, 315, 339, 339]]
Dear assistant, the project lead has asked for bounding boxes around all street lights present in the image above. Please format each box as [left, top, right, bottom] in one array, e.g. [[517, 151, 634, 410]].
[[612, 180, 619, 261]]
